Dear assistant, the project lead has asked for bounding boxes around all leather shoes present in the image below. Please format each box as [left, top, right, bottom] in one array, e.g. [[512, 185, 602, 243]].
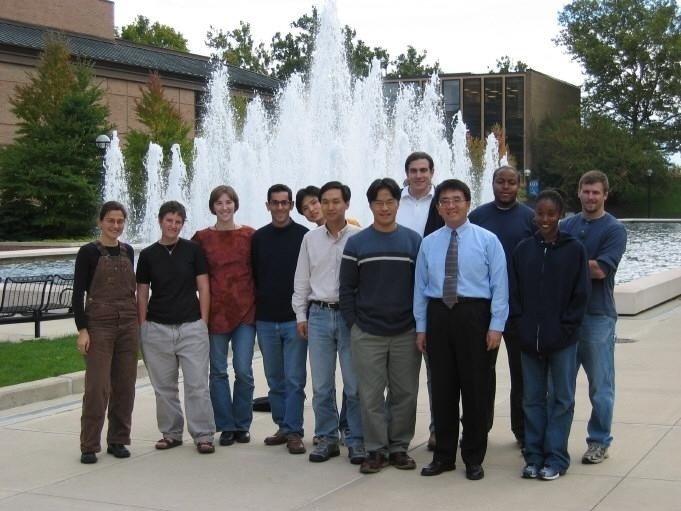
[[106, 444, 130, 459], [81, 453, 97, 465], [196, 441, 216, 453], [264, 429, 486, 479], [155, 438, 183, 450], [234, 430, 250, 443], [220, 431, 234, 446]]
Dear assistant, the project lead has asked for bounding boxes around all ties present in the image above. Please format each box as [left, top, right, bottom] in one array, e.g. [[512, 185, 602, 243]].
[[442, 230, 459, 309]]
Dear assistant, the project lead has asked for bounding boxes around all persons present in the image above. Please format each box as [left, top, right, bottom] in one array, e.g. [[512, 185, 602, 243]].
[[507, 189, 586, 479], [467, 166, 535, 450], [411, 179, 510, 479], [251, 184, 310, 453], [292, 186, 362, 448], [190, 186, 257, 445], [133, 202, 217, 453], [557, 169, 627, 462], [70, 200, 140, 464], [393, 151, 444, 450], [339, 177, 424, 474]]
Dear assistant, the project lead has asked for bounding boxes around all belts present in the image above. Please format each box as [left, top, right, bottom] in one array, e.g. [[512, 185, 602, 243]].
[[309, 299, 338, 310]]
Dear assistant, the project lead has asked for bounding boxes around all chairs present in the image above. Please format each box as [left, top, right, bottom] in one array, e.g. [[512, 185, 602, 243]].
[[0, 273, 54, 340], [25, 273, 74, 342]]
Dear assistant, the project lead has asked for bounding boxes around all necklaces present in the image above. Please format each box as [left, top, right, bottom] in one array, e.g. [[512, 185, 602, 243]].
[[159, 238, 180, 256]]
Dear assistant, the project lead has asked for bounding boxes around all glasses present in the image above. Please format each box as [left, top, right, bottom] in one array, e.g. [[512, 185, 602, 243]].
[[437, 199, 468, 206], [267, 199, 291, 207]]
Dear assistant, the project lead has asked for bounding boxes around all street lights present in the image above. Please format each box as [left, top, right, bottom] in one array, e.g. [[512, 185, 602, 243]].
[[644, 168, 654, 215], [95, 133, 112, 238], [524, 167, 531, 203]]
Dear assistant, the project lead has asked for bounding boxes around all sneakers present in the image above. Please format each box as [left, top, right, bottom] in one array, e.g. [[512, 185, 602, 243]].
[[581, 443, 609, 466], [536, 465, 563, 481], [521, 463, 539, 479]]
